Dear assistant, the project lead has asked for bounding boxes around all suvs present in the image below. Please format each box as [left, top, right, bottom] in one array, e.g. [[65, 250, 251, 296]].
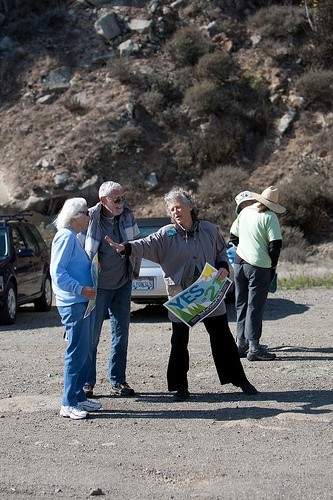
[[0, 212, 54, 325]]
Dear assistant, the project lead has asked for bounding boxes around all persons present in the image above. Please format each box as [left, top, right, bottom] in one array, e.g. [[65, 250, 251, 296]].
[[104, 187, 258, 402], [234, 191, 268, 350], [76, 181, 143, 396], [230, 186, 286, 361], [49, 197, 102, 420]]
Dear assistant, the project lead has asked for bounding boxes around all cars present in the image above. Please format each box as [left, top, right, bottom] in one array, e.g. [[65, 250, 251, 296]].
[[128, 215, 236, 304]]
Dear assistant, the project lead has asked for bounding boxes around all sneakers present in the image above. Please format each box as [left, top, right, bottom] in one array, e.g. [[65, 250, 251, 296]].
[[245, 349, 276, 361], [59, 405, 89, 420], [111, 382, 134, 397], [240, 344, 268, 356], [75, 400, 102, 411], [82, 383, 92, 396]]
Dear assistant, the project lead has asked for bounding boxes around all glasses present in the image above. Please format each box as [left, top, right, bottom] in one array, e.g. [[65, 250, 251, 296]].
[[78, 211, 90, 217], [108, 194, 127, 204]]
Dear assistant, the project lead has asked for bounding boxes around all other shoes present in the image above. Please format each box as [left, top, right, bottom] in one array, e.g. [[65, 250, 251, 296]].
[[232, 378, 258, 394], [172, 390, 191, 402]]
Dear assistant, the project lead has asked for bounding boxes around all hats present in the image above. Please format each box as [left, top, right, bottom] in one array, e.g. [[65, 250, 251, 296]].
[[234, 190, 254, 214], [252, 185, 286, 213]]
